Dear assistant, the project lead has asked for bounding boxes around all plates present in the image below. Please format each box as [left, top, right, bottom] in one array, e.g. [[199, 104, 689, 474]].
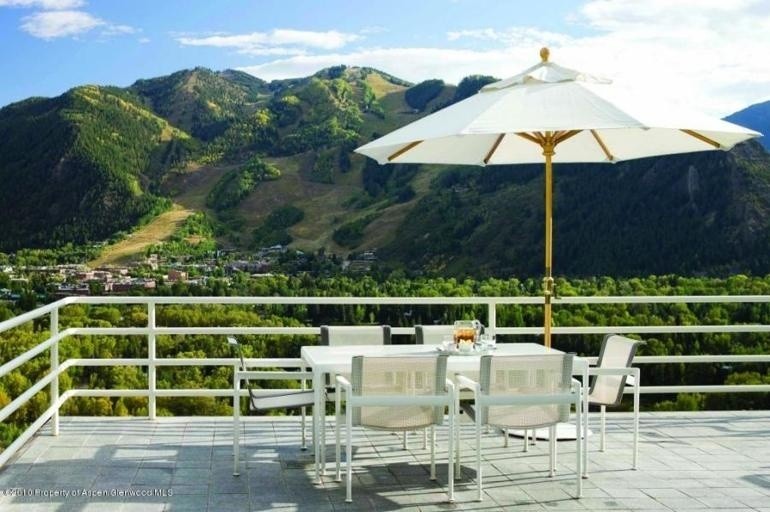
[[437, 343, 485, 355]]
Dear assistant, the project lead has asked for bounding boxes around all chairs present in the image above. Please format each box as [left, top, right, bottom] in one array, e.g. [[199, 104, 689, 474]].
[[225, 321, 642, 506]]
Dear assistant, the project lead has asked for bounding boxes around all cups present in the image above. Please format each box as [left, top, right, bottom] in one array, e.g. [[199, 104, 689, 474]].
[[452, 319, 497, 351]]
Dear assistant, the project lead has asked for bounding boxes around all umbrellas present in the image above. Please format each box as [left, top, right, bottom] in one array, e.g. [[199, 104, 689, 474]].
[[354, 47, 764, 348]]
